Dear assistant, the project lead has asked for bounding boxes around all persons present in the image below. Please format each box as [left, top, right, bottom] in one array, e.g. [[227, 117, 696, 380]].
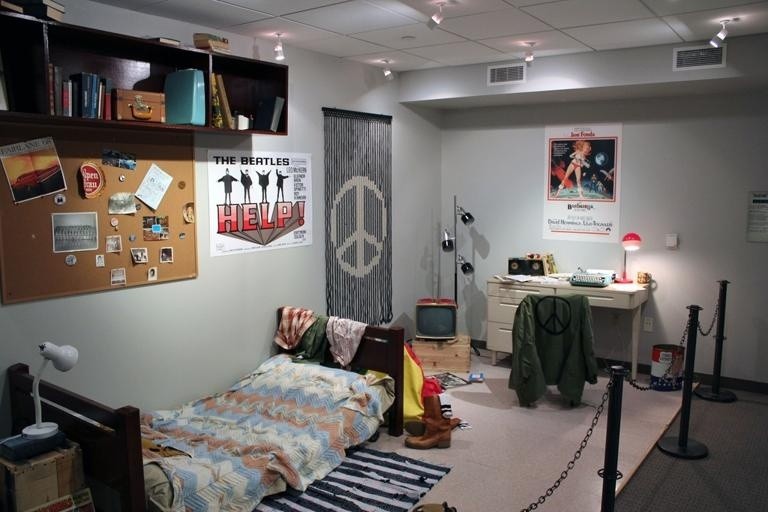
[[215, 163, 290, 203], [554, 140, 592, 198]]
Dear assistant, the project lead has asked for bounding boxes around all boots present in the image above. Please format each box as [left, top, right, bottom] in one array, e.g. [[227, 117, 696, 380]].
[[405, 412, 461, 435], [404, 395, 450, 449]]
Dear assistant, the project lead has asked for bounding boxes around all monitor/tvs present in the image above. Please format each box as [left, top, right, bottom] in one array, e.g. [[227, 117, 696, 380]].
[[416, 298, 458, 339]]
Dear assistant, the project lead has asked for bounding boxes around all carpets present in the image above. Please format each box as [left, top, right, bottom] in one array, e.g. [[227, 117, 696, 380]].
[[249, 446, 453, 512], [601, 380, 766, 512]]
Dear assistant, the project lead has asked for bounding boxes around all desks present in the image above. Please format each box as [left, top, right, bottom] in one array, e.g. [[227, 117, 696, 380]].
[[485, 272, 651, 381]]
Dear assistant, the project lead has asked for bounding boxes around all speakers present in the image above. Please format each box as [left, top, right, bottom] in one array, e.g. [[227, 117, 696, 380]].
[[508, 260, 525, 274], [525, 259, 544, 276]]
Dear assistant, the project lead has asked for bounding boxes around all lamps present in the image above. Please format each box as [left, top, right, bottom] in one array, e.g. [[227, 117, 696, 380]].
[[377, 3, 536, 81], [440, 197, 481, 357], [613, 233, 643, 284], [709, 19, 730, 48], [274, 33, 286, 62], [22, 340, 80, 441]]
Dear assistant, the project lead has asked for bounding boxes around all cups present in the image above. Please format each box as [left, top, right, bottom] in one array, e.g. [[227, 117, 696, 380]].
[[637, 270, 652, 284]]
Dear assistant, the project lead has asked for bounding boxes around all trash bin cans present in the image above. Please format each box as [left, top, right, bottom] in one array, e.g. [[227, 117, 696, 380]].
[[650, 344, 685, 391]]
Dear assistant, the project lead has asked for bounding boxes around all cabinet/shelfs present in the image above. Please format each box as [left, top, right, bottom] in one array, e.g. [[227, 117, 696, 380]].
[[0, 8, 289, 137]]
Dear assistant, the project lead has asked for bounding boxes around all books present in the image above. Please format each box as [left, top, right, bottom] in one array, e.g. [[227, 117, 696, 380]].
[[253, 95, 285, 132], [211, 72, 235, 131], [193, 33, 231, 56], [150, 37, 182, 46], [48, 62, 112, 121], [0, 1, 64, 25]]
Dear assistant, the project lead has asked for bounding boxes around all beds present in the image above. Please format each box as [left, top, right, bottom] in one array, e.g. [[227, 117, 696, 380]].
[[6, 306, 406, 512]]
